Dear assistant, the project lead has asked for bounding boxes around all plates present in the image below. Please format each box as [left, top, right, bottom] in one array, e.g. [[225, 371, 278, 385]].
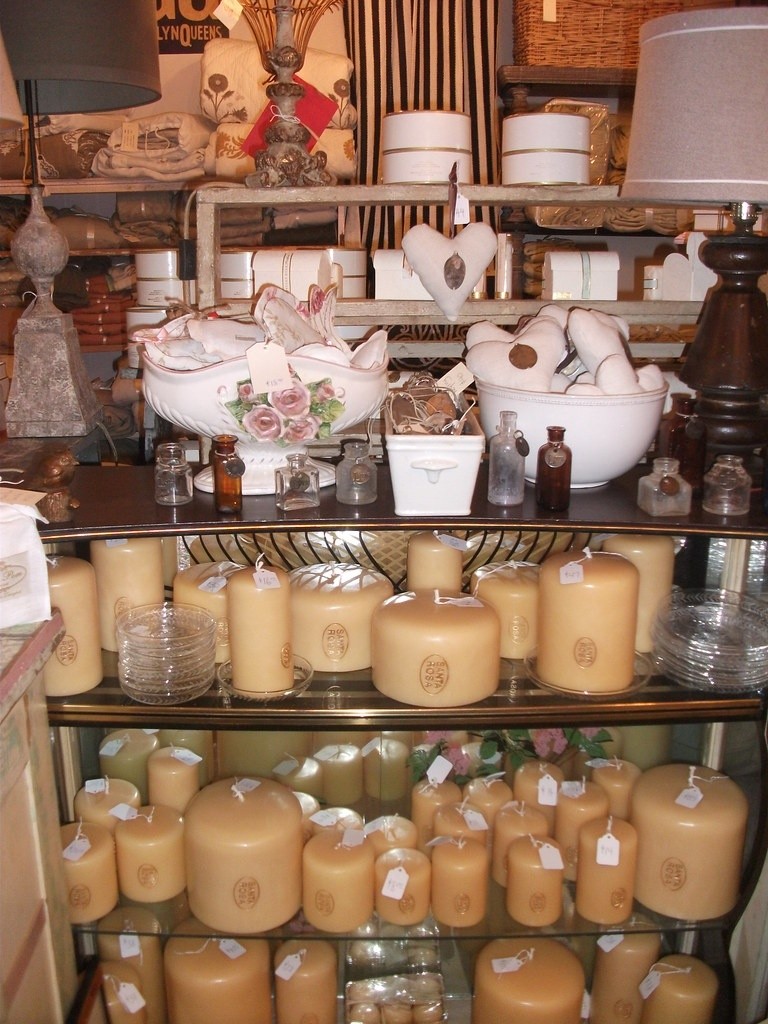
[[648, 587, 768, 693], [216, 652, 314, 706], [113, 602, 216, 705], [522, 645, 653, 702]]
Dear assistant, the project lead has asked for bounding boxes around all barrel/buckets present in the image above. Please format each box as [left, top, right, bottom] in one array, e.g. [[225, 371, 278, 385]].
[[385, 435, 486, 516]]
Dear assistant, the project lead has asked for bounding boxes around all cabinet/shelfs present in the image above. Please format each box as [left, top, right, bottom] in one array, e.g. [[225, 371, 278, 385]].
[[194, 184, 768, 359], [20, 464, 768, 1024]]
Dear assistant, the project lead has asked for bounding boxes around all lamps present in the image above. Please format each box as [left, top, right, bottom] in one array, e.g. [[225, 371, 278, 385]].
[[617, 9, 768, 488], [0, 0, 162, 437]]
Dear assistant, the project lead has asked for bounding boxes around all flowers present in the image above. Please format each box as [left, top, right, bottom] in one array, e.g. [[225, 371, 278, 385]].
[[405, 729, 613, 788], [226, 368, 346, 447]]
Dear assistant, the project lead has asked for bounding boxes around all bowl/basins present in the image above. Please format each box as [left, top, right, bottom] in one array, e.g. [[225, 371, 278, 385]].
[[474, 380, 669, 488]]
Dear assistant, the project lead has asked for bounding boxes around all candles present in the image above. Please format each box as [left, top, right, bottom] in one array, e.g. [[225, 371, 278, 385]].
[[45, 531, 768, 1024]]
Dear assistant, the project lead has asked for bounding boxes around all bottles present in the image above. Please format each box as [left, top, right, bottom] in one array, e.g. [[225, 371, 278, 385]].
[[535, 426, 572, 511], [275, 454, 320, 511], [154, 443, 194, 505], [213, 434, 244, 514], [487, 411, 530, 505], [335, 441, 377, 505], [637, 457, 692, 517], [654, 383, 768, 491], [701, 455, 751, 516]]
